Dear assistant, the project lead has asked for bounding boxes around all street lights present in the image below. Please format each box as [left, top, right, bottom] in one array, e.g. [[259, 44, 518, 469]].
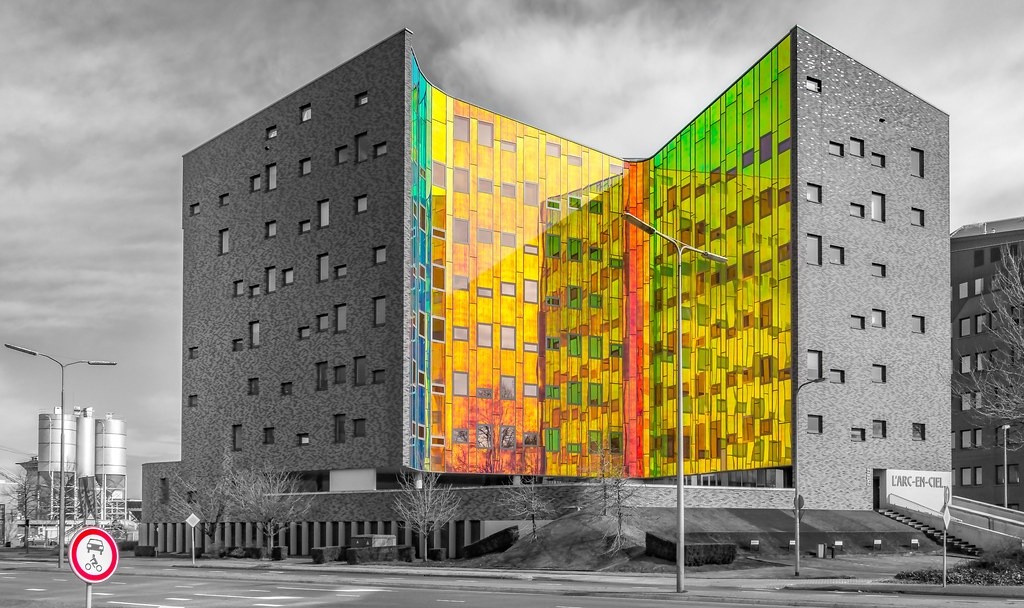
[[619, 212, 730, 593], [1002, 424, 1011, 508], [4, 343, 118, 567], [791, 377, 829, 572]]
